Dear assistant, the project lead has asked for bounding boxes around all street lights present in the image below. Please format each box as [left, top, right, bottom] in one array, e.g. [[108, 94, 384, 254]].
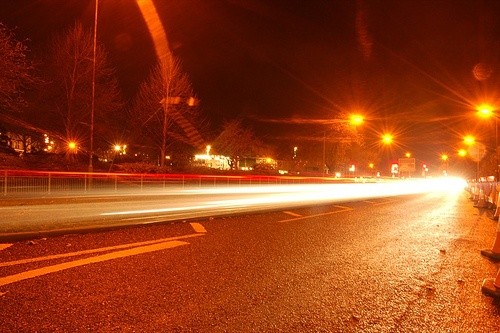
[[321, 114, 360, 177], [478, 103, 499, 184]]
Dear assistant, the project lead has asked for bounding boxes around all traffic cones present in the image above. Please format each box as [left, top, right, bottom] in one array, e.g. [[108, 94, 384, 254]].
[[464, 184, 499, 296]]
